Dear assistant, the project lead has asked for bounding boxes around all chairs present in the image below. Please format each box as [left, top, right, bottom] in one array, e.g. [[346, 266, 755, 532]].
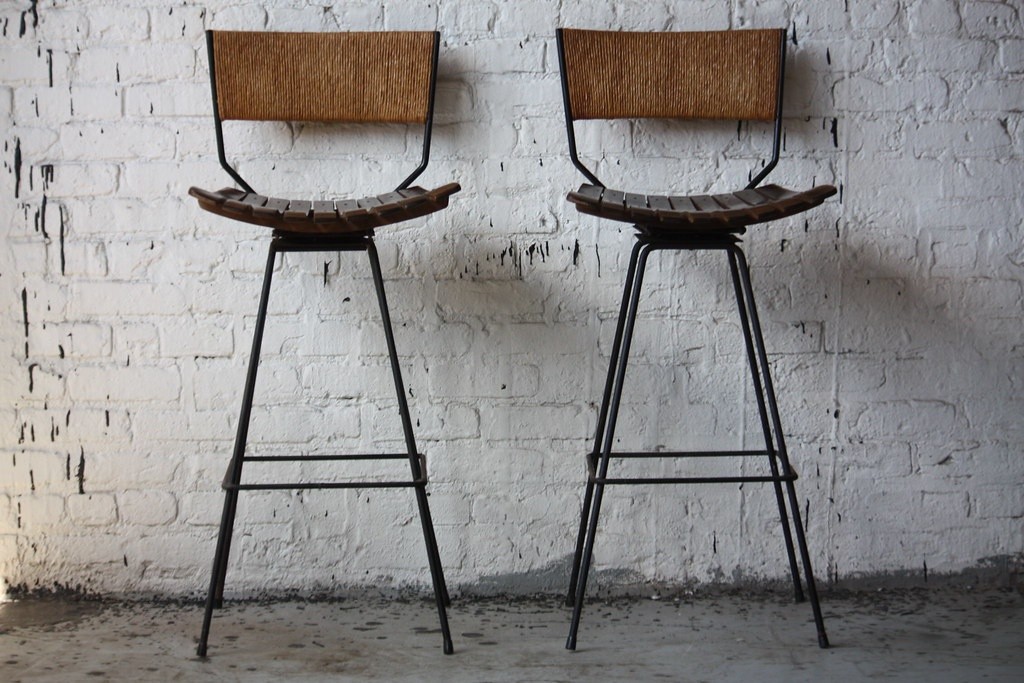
[[188, 30, 461, 657], [555, 27, 837, 650]]
